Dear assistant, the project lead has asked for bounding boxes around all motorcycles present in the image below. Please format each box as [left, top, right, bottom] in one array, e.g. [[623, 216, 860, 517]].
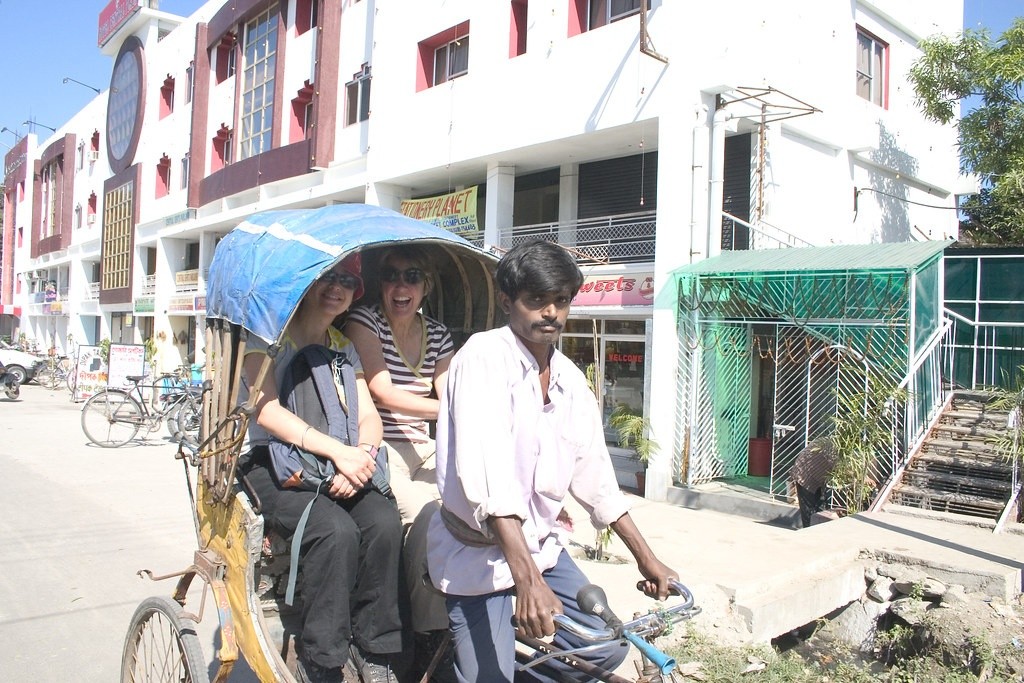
[[0, 362, 20, 399]]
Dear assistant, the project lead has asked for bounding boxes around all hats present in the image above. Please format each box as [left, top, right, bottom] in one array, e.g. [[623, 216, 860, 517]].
[[336, 250, 365, 302]]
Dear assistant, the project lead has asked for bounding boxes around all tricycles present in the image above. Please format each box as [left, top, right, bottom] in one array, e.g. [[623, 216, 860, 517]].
[[119, 201, 701, 682]]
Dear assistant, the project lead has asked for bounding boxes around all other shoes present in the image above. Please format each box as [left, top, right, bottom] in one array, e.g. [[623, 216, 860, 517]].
[[415, 630, 459, 683]]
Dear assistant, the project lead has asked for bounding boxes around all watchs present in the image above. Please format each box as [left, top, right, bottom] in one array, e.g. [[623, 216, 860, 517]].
[[357, 444, 379, 459]]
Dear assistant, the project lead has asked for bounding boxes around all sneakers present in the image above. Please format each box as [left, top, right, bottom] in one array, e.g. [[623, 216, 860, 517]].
[[349, 644, 398, 683], [295, 656, 361, 683]]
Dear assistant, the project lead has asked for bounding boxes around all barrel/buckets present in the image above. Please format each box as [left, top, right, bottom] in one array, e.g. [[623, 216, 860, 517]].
[[749, 438, 771, 477]]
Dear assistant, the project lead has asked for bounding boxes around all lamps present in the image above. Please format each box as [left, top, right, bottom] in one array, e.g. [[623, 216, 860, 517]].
[[22, 120, 56, 133], [1, 127, 24, 139], [63, 77, 101, 95]]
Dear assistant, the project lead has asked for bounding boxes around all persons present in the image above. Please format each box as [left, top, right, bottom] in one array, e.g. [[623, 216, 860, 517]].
[[341, 243, 454, 672], [428, 244, 680, 682], [231, 250, 413, 682]]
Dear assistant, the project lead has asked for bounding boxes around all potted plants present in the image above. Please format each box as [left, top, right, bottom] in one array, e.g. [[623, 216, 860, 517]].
[[796, 357, 921, 528], [608, 403, 662, 493]]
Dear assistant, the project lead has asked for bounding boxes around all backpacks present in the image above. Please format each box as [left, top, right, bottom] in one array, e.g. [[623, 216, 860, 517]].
[[268, 344, 392, 496]]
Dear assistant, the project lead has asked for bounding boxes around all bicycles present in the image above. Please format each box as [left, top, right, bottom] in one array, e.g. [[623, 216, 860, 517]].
[[80, 367, 204, 448], [36, 355, 77, 392]]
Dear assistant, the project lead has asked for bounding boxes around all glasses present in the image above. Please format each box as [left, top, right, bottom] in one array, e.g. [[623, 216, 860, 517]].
[[378, 267, 429, 284], [320, 272, 360, 290]]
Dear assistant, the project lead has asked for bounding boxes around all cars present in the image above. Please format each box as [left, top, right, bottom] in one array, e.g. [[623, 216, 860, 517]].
[[0, 341, 42, 385]]
